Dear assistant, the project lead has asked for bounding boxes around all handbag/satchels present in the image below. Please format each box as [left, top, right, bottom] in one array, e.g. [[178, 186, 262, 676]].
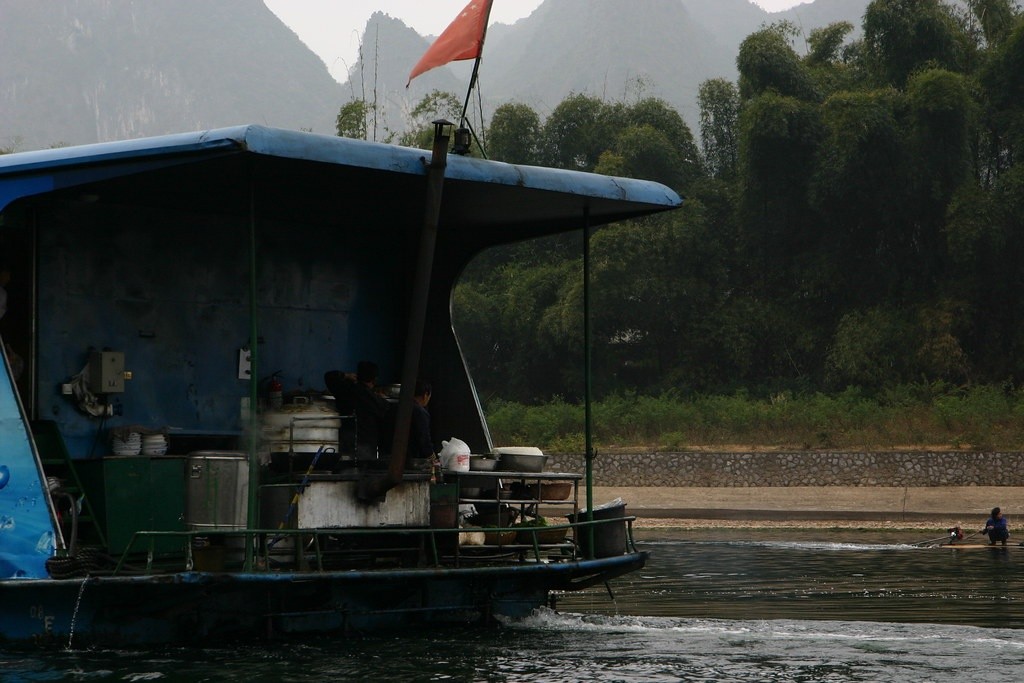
[[439, 437, 471, 472]]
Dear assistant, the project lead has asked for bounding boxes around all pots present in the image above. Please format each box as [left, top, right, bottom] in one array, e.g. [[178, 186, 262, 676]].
[[269, 448, 345, 473]]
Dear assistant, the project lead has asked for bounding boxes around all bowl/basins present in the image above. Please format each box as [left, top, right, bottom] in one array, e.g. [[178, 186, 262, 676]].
[[529, 483, 573, 499], [483, 526, 568, 546], [470, 453, 549, 473], [499, 490, 512, 499]]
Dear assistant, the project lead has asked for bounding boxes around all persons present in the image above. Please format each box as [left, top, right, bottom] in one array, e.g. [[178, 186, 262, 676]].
[[947, 526, 962, 540], [327, 363, 400, 463], [979, 507, 1009, 545], [383, 382, 437, 462]]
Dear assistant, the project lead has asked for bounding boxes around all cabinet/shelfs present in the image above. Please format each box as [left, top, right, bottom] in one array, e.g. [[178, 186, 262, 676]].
[[72, 454, 188, 567]]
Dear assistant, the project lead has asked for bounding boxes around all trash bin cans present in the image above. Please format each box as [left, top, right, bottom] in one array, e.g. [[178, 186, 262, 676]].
[[565, 503, 630, 559]]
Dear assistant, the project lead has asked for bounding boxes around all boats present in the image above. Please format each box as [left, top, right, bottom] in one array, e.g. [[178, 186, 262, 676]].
[[0, 125, 686, 649]]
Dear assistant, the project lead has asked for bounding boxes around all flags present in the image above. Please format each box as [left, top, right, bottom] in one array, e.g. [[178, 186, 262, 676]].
[[405, 0, 489, 89]]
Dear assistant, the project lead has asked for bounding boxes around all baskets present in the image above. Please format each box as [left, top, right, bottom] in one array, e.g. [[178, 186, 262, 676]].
[[519, 528, 569, 545], [485, 531, 516, 545]]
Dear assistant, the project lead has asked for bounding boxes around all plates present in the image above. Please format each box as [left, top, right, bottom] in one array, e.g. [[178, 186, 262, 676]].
[[112, 432, 168, 457]]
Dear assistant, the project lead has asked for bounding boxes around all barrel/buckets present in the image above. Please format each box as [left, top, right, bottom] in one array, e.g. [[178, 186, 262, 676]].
[[184, 450, 249, 530], [565, 504, 625, 558]]
